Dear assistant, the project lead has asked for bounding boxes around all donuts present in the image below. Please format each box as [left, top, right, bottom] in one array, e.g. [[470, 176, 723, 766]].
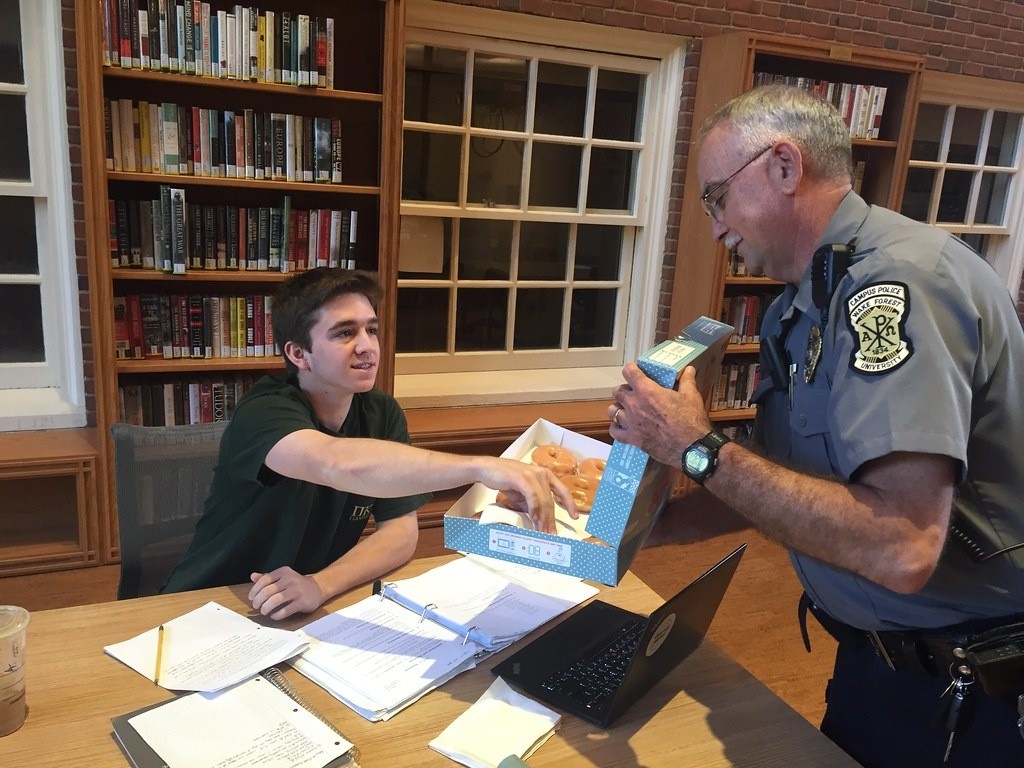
[[470, 444, 613, 547]]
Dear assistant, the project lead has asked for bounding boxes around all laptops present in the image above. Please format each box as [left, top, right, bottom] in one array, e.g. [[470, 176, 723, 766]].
[[490, 543, 747, 728]]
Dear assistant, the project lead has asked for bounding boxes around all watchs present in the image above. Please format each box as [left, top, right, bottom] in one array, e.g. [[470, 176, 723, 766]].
[[681, 429, 730, 483]]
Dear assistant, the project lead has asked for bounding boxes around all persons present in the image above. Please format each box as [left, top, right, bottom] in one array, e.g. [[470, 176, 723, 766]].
[[160, 267, 581, 623], [606, 85, 1022, 768]]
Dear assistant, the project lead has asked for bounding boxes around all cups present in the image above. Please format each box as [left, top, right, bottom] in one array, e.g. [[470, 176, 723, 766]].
[[0, 604, 30, 738]]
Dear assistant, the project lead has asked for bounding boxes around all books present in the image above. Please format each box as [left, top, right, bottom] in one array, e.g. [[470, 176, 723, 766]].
[[113, 295, 285, 358], [108, 180, 358, 273], [120, 373, 269, 426], [103, 96, 342, 184], [715, 421, 753, 447], [754, 71, 889, 144], [721, 294, 775, 344], [727, 157, 867, 277], [98, 0, 334, 92], [707, 361, 764, 413], [108, 668, 363, 768], [282, 554, 567, 724]]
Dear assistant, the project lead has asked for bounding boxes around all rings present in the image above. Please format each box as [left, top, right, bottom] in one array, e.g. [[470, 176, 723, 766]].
[[614, 408, 622, 418]]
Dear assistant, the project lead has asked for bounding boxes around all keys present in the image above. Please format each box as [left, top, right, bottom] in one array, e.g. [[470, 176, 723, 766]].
[[942, 684, 970, 763]]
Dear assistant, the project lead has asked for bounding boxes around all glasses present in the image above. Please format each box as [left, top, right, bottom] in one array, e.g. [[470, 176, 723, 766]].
[[699, 145, 774, 221]]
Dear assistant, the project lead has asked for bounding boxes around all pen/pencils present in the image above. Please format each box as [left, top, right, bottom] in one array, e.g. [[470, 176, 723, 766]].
[[154, 624, 165, 683]]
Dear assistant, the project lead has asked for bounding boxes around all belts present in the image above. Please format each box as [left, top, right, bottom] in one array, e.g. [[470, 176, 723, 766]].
[[808, 601, 937, 677]]
